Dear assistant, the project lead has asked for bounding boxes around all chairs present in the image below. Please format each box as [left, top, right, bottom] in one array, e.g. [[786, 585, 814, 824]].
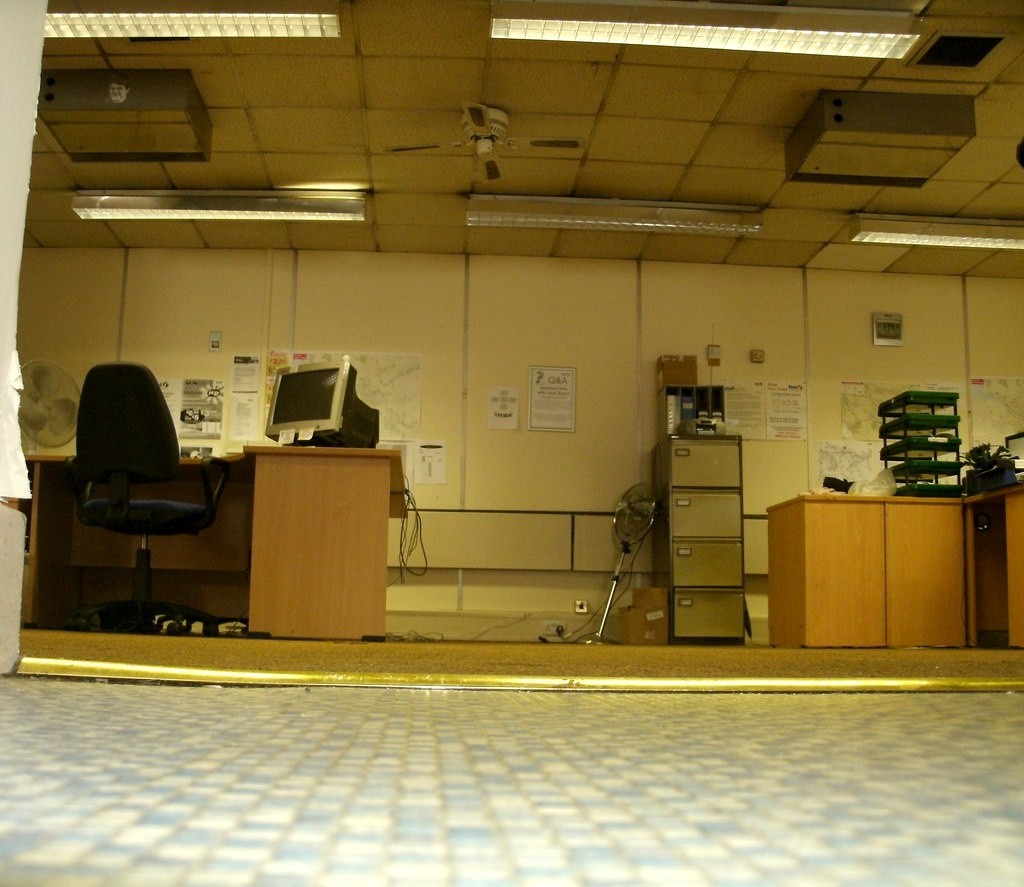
[[62, 361, 230, 636]]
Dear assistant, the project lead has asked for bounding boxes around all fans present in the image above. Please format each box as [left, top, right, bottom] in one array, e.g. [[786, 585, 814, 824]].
[[384, 102, 583, 183], [18, 360, 82, 455], [556, 482, 659, 645]]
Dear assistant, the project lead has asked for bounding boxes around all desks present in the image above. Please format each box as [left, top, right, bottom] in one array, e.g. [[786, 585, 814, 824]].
[[964, 485, 1024, 649]]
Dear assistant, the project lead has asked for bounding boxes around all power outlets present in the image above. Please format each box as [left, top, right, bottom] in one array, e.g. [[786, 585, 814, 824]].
[[575, 600, 587, 613], [541, 621, 565, 635]]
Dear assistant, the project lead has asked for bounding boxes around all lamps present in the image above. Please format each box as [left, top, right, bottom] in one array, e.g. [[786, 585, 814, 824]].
[[466, 194, 763, 234], [849, 213, 1024, 250], [489, 0, 925, 59], [43, 1, 340, 37], [73, 190, 368, 221]]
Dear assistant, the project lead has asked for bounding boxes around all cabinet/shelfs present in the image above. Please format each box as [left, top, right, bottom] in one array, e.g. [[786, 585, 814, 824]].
[[766, 496, 965, 649], [653, 435, 752, 646], [879, 390, 965, 497], [0, 445, 408, 642]]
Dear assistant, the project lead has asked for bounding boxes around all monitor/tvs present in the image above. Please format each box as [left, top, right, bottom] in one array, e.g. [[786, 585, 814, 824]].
[[264, 358, 380, 449]]
